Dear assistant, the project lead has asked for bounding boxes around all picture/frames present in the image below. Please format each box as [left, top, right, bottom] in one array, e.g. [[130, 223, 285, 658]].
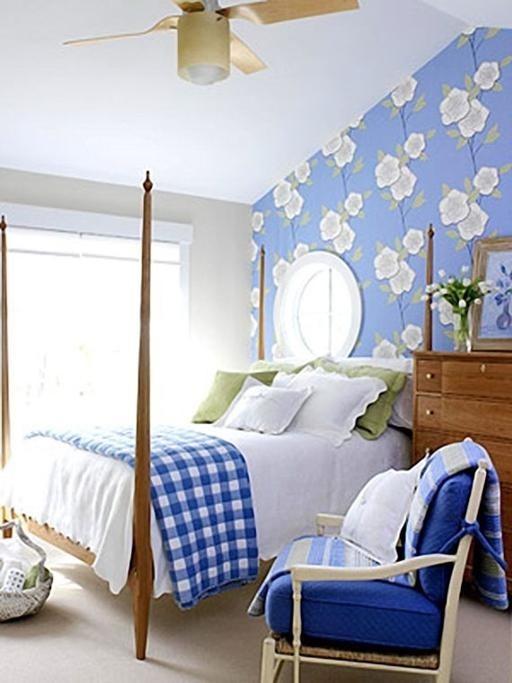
[[469, 235, 512, 349]]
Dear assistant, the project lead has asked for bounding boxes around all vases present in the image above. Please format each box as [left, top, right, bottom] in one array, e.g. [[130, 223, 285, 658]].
[[451, 310, 472, 350]]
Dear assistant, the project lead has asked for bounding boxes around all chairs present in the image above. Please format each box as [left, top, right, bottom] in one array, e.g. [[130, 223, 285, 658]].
[[257, 435, 489, 683]]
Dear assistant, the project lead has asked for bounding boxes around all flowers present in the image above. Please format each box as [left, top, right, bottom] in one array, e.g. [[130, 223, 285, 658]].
[[419, 263, 500, 337]]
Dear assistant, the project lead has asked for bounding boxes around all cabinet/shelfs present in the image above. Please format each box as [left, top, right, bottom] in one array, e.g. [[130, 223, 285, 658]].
[[411, 349, 511, 596]]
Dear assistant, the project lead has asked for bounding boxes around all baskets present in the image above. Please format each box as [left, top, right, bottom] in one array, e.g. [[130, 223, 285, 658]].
[[1, 518, 56, 619]]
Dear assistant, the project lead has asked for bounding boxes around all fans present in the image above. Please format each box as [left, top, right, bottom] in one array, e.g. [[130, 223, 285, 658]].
[[64, 0, 359, 83]]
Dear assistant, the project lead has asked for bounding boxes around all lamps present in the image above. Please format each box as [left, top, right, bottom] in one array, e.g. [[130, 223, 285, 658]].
[[178, 11, 226, 82]]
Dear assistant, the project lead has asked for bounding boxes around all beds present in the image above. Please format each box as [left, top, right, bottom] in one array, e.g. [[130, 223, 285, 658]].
[[0, 156, 435, 657]]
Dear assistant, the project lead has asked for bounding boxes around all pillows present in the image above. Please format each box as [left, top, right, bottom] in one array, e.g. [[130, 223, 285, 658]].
[[315, 355, 410, 440], [337, 452, 431, 565], [214, 375, 312, 435], [192, 368, 278, 423], [286, 365, 389, 446], [250, 359, 316, 376], [325, 355, 411, 430]]
